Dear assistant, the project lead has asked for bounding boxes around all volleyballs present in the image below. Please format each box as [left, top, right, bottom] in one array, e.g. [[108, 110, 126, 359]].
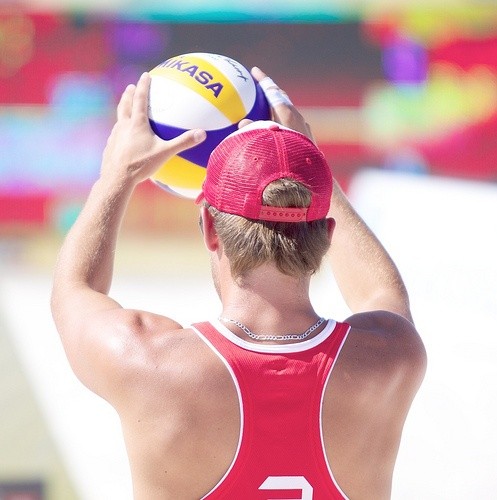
[[147, 52, 272, 199]]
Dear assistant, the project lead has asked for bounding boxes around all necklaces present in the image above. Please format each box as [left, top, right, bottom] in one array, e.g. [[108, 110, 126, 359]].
[[214, 315, 326, 341]]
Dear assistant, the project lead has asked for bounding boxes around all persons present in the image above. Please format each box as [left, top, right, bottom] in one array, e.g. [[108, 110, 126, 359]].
[[50, 51, 427, 500]]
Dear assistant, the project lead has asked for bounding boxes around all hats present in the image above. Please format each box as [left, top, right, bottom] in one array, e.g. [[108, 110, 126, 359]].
[[195, 120, 333, 222]]
